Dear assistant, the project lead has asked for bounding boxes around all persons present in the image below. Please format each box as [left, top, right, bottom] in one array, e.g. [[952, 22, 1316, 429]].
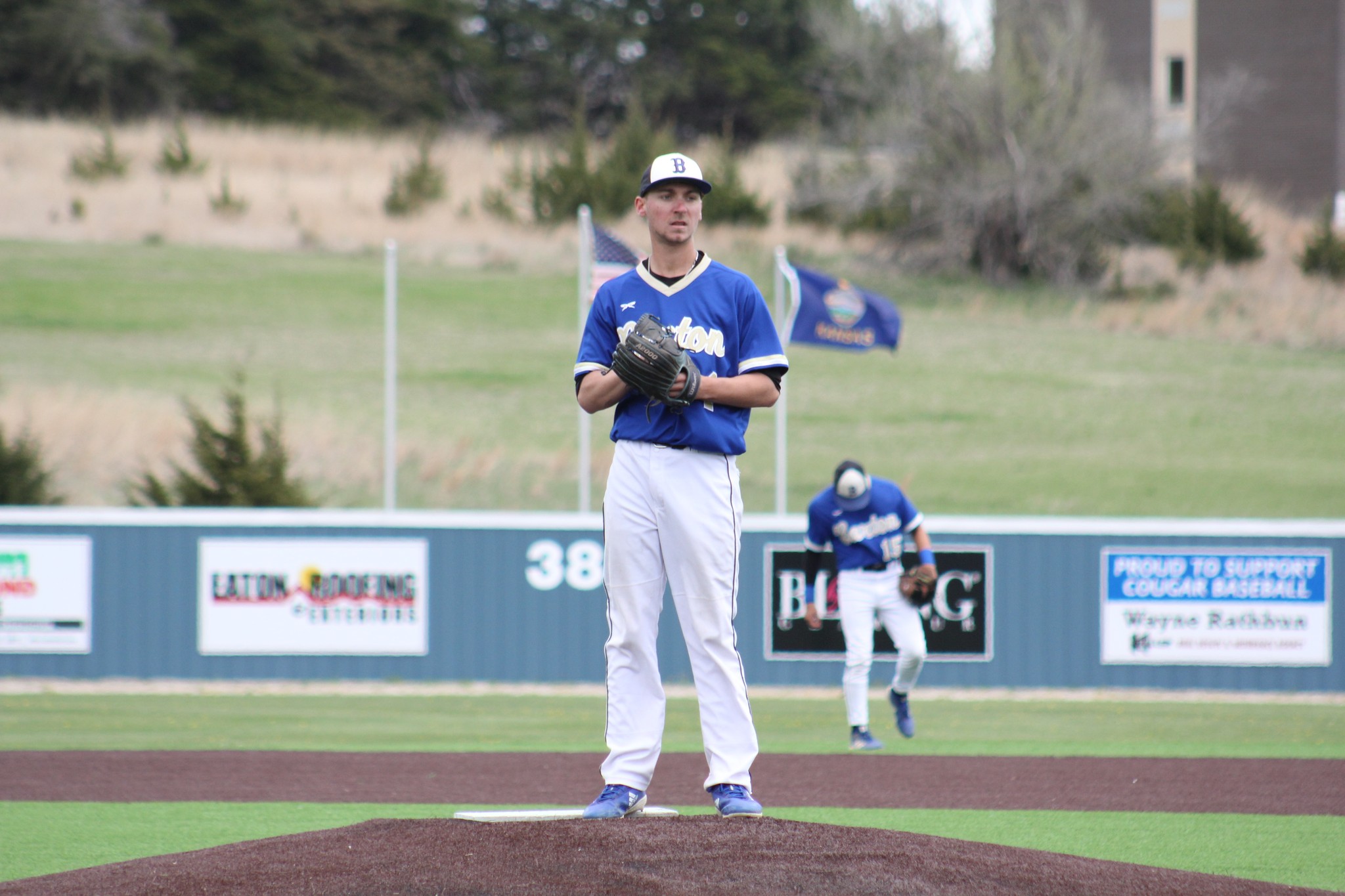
[[573, 152, 790, 822], [804, 459, 940, 752]]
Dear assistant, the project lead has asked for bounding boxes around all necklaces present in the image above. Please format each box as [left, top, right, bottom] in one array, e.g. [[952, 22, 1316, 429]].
[[645, 249, 699, 280]]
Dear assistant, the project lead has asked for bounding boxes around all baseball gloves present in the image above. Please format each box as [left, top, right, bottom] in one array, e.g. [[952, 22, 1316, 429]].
[[611, 313, 701, 415], [900, 565, 938, 609]]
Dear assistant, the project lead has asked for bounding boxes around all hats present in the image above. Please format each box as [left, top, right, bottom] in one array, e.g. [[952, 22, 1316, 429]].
[[833, 461, 871, 511], [639, 152, 712, 198]]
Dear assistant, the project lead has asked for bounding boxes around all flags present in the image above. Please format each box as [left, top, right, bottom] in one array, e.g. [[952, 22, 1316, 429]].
[[587, 220, 649, 306], [782, 260, 904, 354]]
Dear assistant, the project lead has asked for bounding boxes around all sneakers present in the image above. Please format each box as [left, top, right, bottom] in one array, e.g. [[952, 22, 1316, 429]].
[[581, 784, 647, 820], [711, 783, 763, 818], [849, 727, 885, 751], [890, 688, 915, 738]]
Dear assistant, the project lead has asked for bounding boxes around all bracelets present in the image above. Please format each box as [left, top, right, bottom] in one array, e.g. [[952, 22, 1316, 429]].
[[920, 550, 936, 566]]
